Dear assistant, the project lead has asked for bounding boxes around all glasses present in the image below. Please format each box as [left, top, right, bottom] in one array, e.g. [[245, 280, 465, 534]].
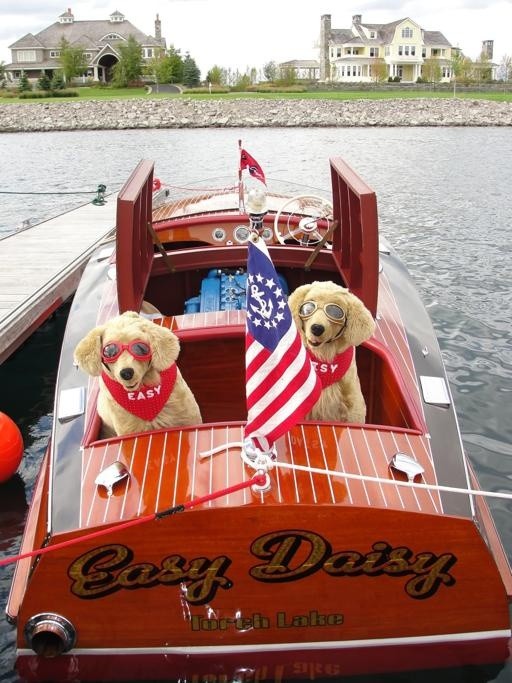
[[298, 300, 346, 325], [101, 340, 151, 363]]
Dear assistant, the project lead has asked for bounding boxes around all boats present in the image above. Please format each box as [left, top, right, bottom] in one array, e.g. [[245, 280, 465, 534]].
[[0, 143, 510, 679]]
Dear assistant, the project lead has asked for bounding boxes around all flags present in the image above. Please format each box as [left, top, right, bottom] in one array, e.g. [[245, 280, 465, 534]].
[[239, 148, 268, 187], [243, 235, 323, 459]]
